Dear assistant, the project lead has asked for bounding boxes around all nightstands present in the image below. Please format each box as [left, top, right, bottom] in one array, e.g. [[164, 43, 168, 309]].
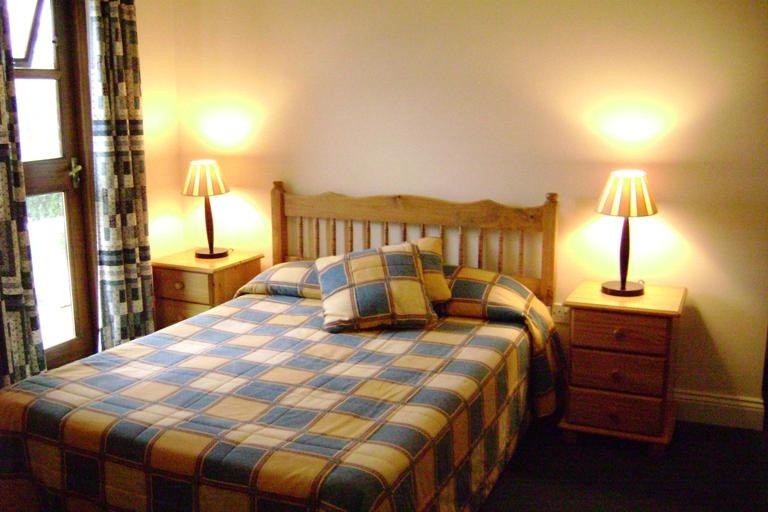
[[150, 249, 264, 325], [565, 282, 688, 443]]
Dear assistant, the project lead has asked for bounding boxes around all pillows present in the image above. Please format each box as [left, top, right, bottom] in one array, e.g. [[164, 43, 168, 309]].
[[316, 227, 453, 337]]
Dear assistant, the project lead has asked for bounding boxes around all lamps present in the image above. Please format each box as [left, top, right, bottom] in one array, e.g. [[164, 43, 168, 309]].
[[598, 169, 654, 296], [184, 161, 232, 258]]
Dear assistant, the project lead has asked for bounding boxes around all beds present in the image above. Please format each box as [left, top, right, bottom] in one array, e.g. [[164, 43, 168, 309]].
[[0, 185, 556, 510]]
